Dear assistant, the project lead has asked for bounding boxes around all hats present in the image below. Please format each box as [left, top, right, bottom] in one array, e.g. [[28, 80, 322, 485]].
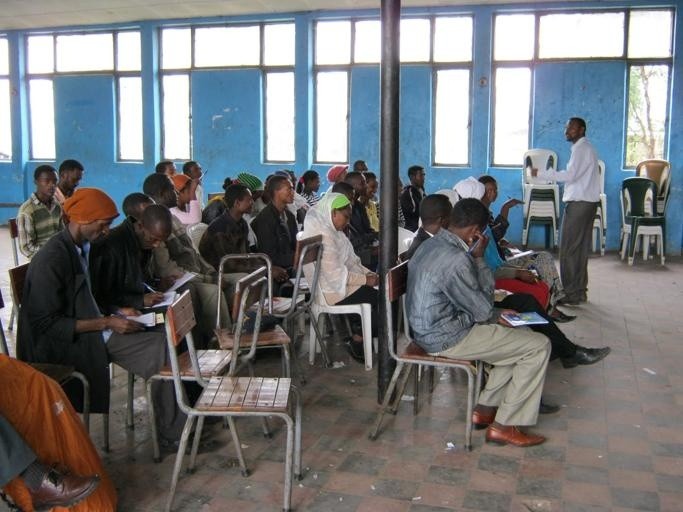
[[327, 166, 348, 182]]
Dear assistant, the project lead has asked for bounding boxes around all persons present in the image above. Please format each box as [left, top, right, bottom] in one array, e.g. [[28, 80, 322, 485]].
[[0, 159, 212, 512], [530, 118, 601, 307], [198, 160, 427, 365], [407, 176, 611, 447]]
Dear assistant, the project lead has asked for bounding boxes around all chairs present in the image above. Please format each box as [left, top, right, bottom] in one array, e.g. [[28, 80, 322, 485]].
[[0, 290, 91, 436], [10, 263, 133, 450], [616, 160, 671, 266], [6, 217, 34, 331], [522, 149, 559, 250], [592, 161, 606, 255], [368, 260, 475, 452], [396, 252, 434, 417]]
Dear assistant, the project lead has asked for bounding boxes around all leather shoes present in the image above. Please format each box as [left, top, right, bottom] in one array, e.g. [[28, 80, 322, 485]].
[[552, 310, 577, 322], [557, 295, 579, 305], [28, 462, 101, 512], [539, 400, 560, 414], [472, 405, 497, 429], [560, 345, 610, 369], [343, 337, 365, 364], [204, 416, 223, 423], [580, 291, 587, 301], [485, 424, 545, 447], [162, 438, 218, 454]]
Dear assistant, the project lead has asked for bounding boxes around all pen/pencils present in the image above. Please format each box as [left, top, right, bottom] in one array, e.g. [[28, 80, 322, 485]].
[[506, 195, 519, 209], [141, 281, 165, 302], [468, 227, 489, 254], [199, 168, 208, 180], [527, 270, 539, 285]]
